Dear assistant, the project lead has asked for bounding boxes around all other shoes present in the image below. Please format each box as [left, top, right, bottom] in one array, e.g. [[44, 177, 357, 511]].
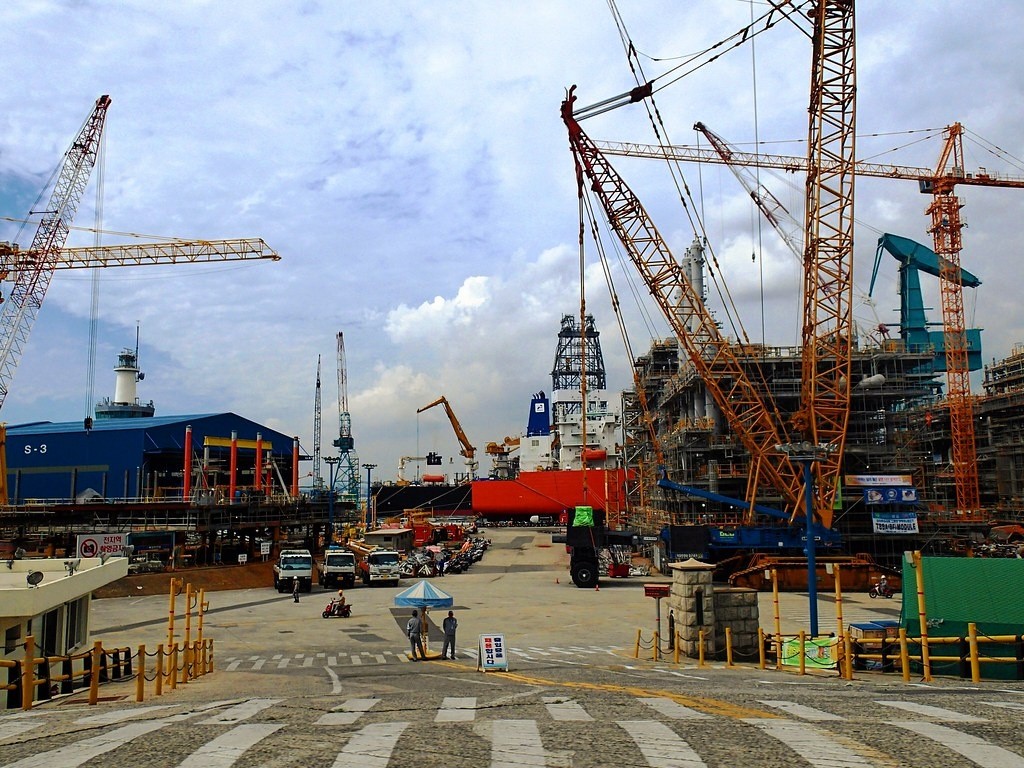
[[414, 660, 417, 662], [442, 657, 446, 660], [422, 658, 428, 661], [294, 601, 299, 602], [451, 656, 455, 660]]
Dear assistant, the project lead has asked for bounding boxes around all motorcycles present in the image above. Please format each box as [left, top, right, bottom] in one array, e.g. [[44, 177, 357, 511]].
[[870, 583, 894, 599]]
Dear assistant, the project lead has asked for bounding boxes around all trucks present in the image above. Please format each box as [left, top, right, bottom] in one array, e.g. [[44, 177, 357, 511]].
[[359, 552, 400, 585], [318, 553, 356, 588], [273, 550, 313, 593]]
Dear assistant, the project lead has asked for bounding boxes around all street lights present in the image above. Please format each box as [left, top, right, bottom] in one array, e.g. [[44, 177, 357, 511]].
[[363, 464, 377, 530], [323, 456, 341, 538]]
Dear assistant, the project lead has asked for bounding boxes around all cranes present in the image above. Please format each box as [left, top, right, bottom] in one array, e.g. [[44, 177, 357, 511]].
[[585, 123, 1022, 523], [554, 0, 860, 532], [0, 215, 281, 276], [0, 92, 111, 412]]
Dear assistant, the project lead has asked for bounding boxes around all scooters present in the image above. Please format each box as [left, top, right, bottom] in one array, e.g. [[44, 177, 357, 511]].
[[322, 597, 353, 618], [444, 536, 488, 574]]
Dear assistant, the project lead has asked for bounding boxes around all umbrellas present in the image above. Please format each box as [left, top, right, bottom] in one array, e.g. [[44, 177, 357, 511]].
[[394, 580, 453, 654]]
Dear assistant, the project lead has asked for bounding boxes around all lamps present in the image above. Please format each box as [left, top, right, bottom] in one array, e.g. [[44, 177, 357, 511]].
[[26, 569, 45, 589], [120, 544, 134, 558], [63, 558, 81, 577], [97, 550, 111, 566], [15, 546, 26, 559]]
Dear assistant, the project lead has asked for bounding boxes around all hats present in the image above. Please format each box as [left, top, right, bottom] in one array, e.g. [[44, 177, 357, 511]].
[[293, 576, 297, 579], [881, 575, 886, 579], [338, 590, 342, 594]]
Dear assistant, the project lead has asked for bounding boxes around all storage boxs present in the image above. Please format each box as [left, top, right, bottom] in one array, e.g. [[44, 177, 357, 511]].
[[848, 620, 902, 672]]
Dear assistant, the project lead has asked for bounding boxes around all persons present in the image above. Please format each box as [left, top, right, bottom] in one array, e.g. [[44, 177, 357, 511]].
[[441, 611, 457, 660], [879, 575, 887, 594], [331, 590, 345, 614], [407, 610, 428, 662], [292, 576, 300, 603], [439, 559, 444, 577]]
